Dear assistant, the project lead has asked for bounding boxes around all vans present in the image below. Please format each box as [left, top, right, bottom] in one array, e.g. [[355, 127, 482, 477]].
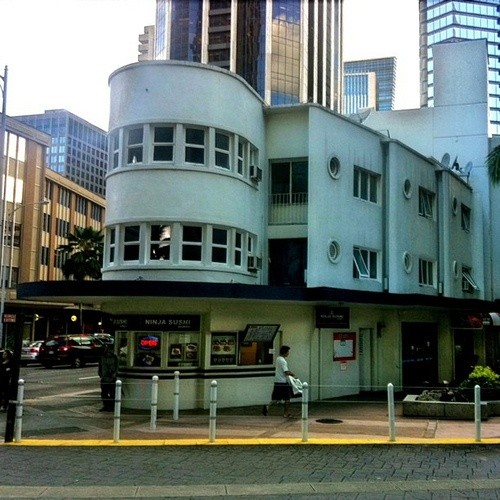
[[37, 336, 112, 370]]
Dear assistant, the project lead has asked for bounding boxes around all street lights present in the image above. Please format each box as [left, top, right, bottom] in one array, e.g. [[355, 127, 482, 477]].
[[0, 200, 50, 350]]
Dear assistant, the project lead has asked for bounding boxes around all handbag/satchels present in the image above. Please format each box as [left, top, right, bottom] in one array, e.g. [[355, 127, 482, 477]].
[[287, 376, 303, 398]]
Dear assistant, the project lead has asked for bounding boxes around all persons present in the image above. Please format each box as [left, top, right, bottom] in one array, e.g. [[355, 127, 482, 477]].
[[0, 349, 12, 410], [263, 346, 295, 418], [98, 345, 118, 411]]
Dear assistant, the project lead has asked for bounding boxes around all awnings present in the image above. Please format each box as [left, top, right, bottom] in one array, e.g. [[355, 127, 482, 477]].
[[454, 313, 500, 325]]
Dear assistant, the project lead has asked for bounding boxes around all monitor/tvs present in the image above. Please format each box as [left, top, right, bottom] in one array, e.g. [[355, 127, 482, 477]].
[[316, 306, 350, 329]]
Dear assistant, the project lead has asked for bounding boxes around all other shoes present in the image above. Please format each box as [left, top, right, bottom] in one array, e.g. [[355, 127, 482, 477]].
[[283, 414, 296, 419], [99, 407, 114, 412], [263, 405, 269, 417], [1, 409, 8, 413]]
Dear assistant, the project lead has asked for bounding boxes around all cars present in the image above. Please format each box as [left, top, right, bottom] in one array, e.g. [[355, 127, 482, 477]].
[[20, 340, 44, 367]]
